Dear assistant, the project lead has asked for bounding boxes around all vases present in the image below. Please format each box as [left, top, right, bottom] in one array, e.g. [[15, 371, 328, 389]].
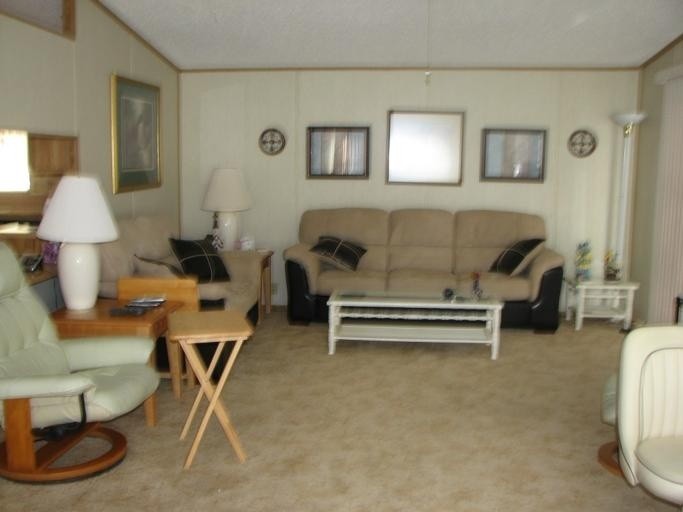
[[575, 267, 618, 283]]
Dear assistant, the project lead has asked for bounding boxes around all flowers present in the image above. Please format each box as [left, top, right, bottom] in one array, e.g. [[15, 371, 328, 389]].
[[573, 244, 622, 273]]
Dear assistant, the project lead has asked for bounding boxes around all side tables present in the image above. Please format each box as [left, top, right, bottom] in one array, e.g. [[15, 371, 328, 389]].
[[47, 297, 184, 427], [563, 278, 641, 332], [252, 248, 273, 325]]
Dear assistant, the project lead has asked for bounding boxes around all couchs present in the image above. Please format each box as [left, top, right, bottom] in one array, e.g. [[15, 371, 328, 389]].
[[98, 215, 260, 389], [280, 206, 564, 334]]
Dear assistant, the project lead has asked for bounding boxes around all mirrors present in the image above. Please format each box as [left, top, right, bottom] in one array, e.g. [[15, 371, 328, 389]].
[[0, 132, 83, 286]]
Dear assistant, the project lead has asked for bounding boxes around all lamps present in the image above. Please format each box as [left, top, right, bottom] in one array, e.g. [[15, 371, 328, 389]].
[[200, 168, 253, 252], [33, 171, 120, 311], [604, 111, 646, 307]]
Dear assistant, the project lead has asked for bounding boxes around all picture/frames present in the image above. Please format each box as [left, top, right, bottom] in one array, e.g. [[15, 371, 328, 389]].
[[107, 74, 161, 197], [303, 110, 547, 189]]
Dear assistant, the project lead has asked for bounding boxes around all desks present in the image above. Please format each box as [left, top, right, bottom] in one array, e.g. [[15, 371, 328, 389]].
[[164, 306, 252, 471]]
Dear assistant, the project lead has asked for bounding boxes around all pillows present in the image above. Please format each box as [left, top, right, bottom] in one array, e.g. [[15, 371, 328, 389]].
[[308, 231, 368, 273], [489, 237, 548, 277], [130, 234, 230, 286]]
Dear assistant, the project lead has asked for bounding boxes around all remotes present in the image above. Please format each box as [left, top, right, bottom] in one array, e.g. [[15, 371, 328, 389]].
[[110, 297, 167, 316]]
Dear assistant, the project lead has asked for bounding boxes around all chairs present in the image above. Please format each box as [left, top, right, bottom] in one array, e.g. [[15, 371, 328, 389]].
[[0, 243, 160, 483], [616, 322, 683, 507]]
